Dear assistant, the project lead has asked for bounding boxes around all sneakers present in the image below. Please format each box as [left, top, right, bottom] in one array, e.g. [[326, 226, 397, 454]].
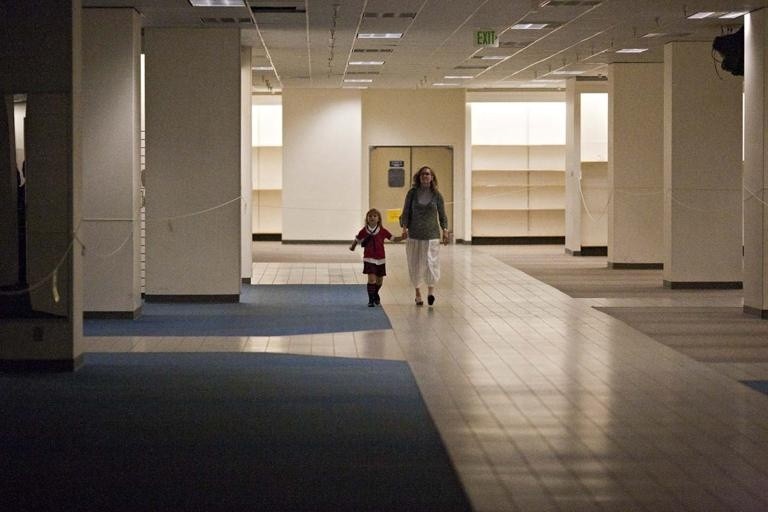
[[374, 295, 379, 305], [367, 302, 375, 307], [427, 295, 434, 305], [414, 297, 422, 305]]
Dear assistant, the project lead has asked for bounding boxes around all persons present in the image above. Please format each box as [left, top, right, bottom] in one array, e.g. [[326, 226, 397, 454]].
[[400, 166, 450, 306], [349, 207, 407, 307]]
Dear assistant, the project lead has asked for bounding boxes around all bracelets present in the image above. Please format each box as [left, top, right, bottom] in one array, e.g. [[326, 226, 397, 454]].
[[443, 228, 448, 231]]
